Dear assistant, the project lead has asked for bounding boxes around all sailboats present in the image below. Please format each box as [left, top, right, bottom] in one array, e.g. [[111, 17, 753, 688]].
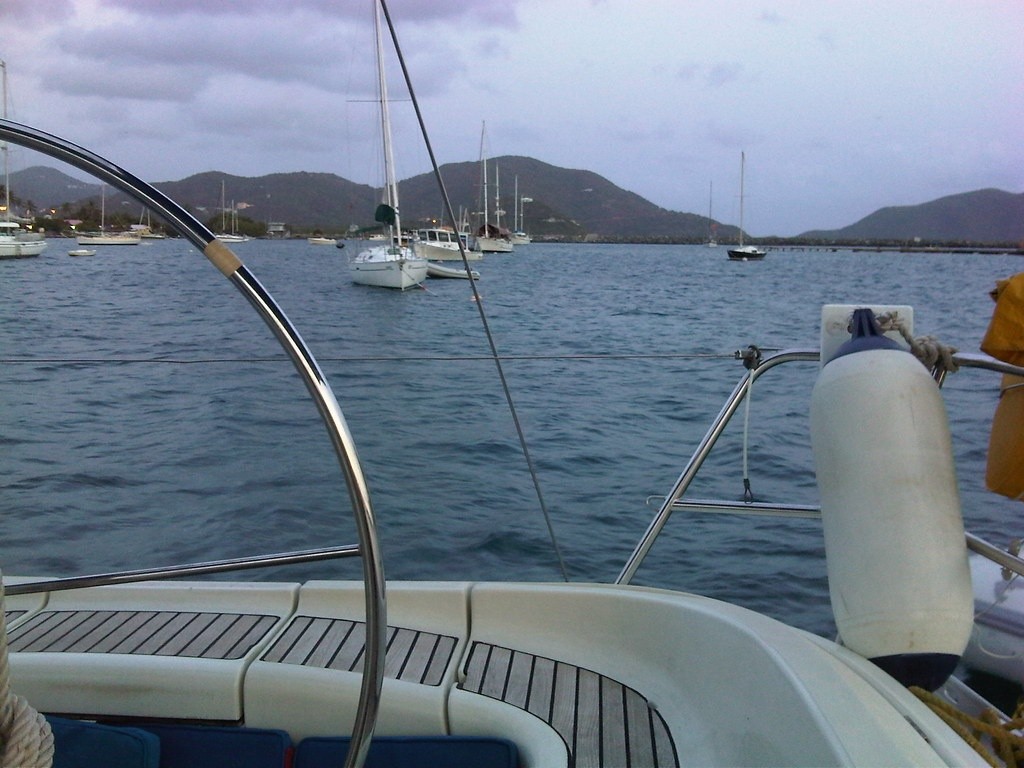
[[507, 173, 531, 245], [348, 0, 481, 292], [213, 178, 257, 242], [469, 118, 514, 252], [727, 150, 768, 260], [139, 206, 166, 239], [75, 181, 142, 245], [0, 60, 50, 258]]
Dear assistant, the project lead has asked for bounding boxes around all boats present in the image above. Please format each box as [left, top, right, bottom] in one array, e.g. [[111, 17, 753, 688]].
[[67, 249, 97, 257], [404, 228, 483, 262], [307, 237, 337, 244]]
